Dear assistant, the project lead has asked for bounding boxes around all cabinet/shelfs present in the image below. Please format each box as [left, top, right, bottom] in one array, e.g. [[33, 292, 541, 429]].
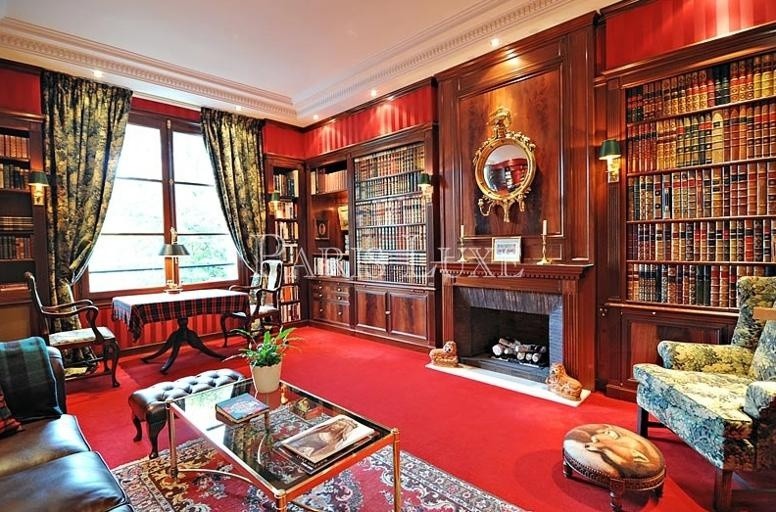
[[0, 110, 52, 342]]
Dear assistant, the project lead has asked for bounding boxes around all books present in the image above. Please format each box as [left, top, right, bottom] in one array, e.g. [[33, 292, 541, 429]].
[[215, 393, 270, 425], [0, 129, 36, 293], [353, 140, 428, 286], [270, 414, 380, 476], [625, 47, 776, 309], [307, 158, 350, 279], [273, 167, 303, 324]]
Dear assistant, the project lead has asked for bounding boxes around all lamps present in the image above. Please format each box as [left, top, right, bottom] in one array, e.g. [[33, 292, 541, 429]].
[[158, 243, 190, 293], [26, 170, 50, 206]]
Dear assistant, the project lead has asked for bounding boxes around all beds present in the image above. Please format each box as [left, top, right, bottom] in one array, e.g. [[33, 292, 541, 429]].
[[164, 378, 403, 512]]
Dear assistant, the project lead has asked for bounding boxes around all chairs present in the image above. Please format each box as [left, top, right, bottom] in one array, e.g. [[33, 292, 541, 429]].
[[632, 276, 776, 512], [24, 271, 120, 388], [220, 259, 290, 349]]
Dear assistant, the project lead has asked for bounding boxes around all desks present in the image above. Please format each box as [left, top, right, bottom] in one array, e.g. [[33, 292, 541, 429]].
[[112, 289, 249, 374]]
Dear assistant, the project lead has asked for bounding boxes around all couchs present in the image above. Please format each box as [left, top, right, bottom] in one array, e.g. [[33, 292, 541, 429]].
[[0, 337, 134, 512]]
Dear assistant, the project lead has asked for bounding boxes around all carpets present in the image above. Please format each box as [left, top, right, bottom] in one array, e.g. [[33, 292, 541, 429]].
[[111, 437, 529, 512]]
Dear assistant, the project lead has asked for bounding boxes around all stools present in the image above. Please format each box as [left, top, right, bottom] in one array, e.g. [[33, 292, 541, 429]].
[[128, 367, 247, 459], [561, 423, 666, 511]]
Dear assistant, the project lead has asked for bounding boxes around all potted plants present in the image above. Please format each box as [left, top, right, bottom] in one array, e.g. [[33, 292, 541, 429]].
[[229, 321, 304, 394]]
